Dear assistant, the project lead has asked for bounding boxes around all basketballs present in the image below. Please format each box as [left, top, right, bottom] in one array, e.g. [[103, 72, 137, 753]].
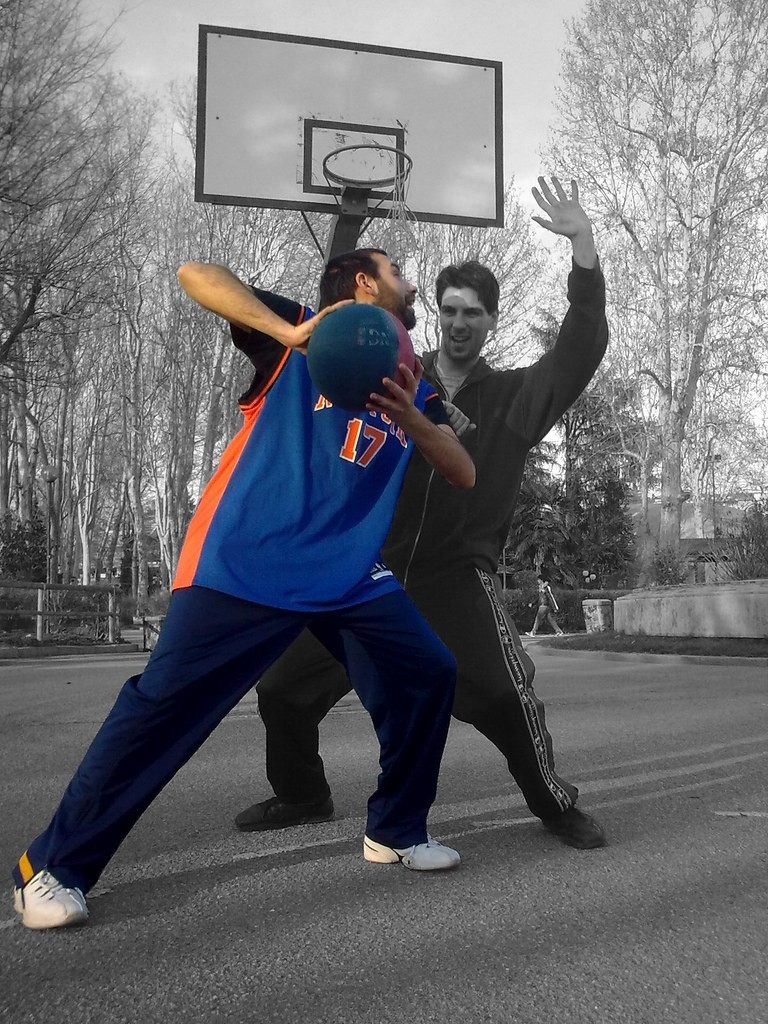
[[305, 302, 417, 413]]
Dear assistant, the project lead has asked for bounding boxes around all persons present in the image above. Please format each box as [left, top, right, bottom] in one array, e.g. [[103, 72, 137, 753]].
[[15, 248, 474, 929], [236, 177, 612, 850]]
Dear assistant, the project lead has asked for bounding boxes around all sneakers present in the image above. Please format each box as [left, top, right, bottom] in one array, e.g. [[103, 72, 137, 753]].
[[543, 806, 605, 849], [363, 831, 460, 870], [233, 792, 333, 832], [15, 866, 90, 928]]
